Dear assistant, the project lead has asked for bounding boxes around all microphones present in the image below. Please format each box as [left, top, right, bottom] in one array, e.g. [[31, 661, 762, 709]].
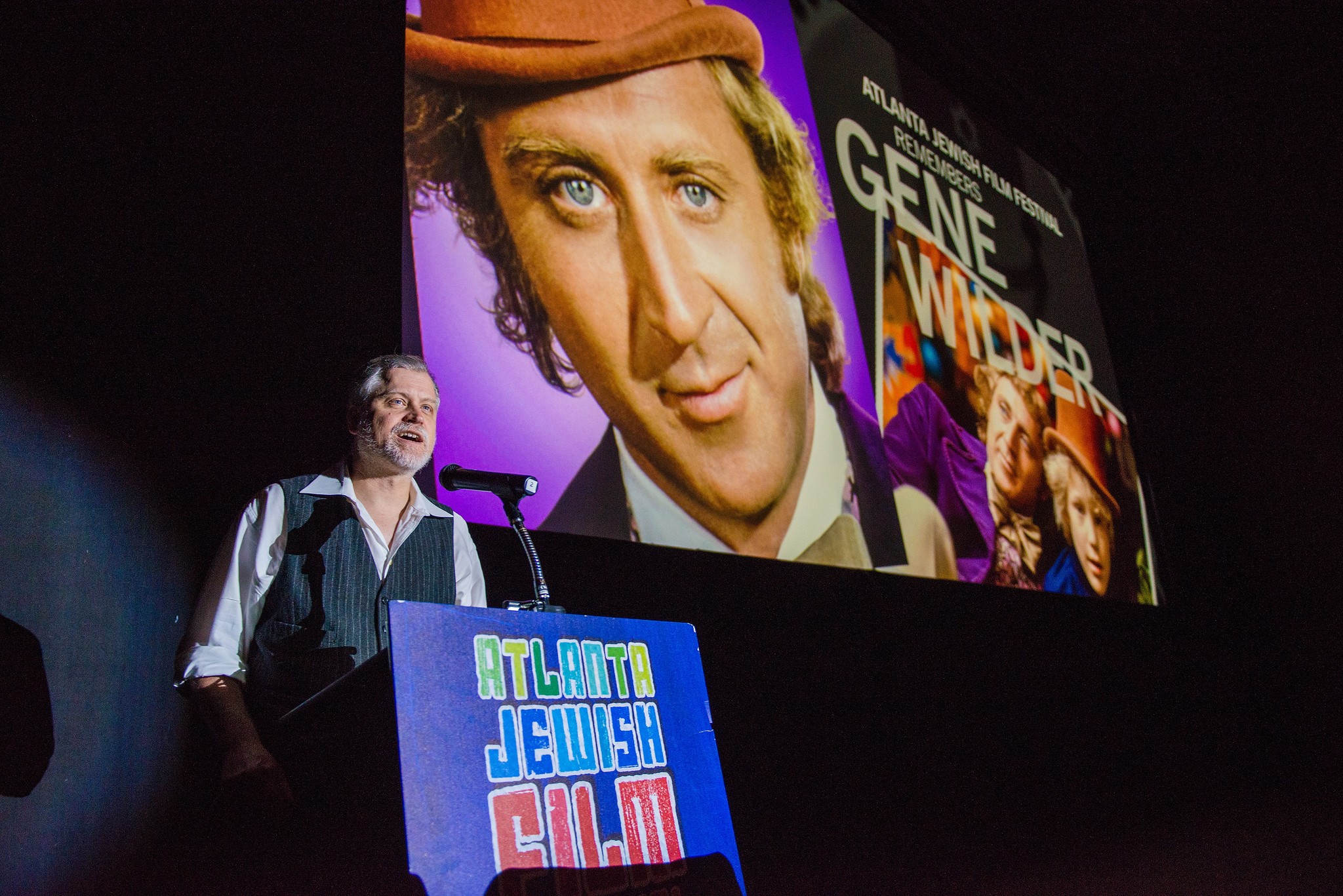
[[438, 463, 538, 497]]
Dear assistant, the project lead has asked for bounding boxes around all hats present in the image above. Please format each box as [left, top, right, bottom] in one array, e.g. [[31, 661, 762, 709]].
[[1041, 370, 1123, 518], [405, 1, 764, 85]]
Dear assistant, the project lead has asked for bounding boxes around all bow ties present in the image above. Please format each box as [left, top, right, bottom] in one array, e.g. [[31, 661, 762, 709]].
[[988, 487, 1043, 575]]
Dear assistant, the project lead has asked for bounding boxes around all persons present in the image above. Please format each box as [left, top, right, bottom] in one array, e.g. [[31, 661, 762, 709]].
[[883, 362, 1051, 586], [174, 354, 489, 820], [404, 0, 959, 584], [1043, 368, 1138, 598]]
